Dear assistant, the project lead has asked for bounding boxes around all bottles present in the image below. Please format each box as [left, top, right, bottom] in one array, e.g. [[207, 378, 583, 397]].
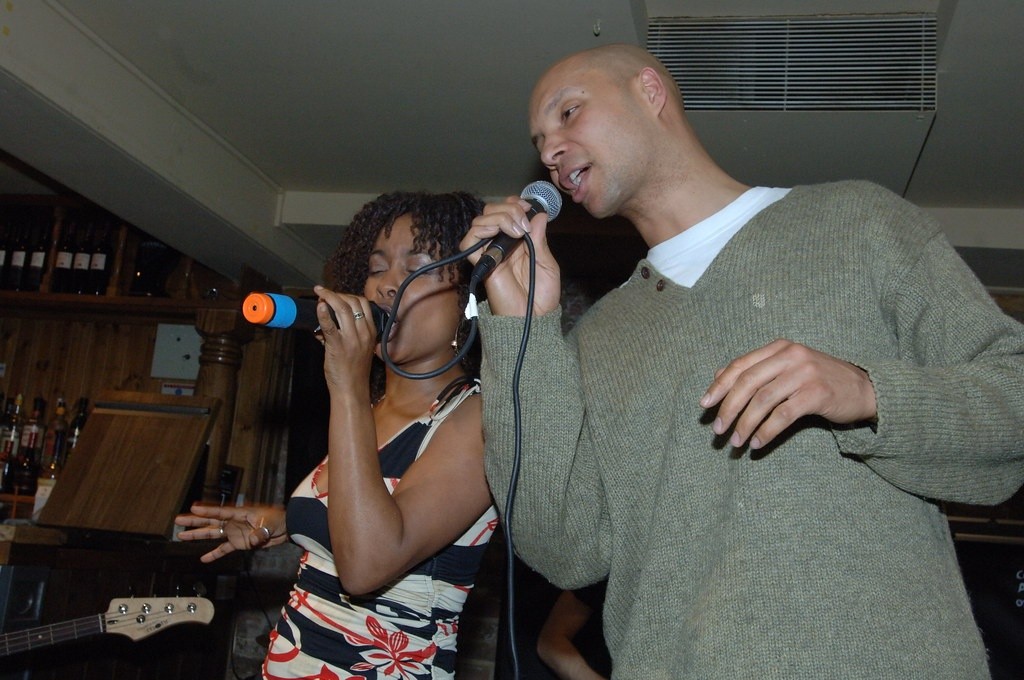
[[0, 214, 163, 298], [0, 393, 89, 495]]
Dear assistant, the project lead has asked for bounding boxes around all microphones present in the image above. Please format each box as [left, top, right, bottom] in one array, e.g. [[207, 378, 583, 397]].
[[472, 180, 562, 282], [242, 292, 389, 344]]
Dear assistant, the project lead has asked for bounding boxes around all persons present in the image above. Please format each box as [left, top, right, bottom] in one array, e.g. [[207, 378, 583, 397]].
[[537, 573, 612, 680], [174, 191, 501, 680], [459, 44, 1024, 680]]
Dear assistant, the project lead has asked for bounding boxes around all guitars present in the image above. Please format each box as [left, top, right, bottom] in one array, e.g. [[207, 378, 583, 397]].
[[0, 582, 215, 657]]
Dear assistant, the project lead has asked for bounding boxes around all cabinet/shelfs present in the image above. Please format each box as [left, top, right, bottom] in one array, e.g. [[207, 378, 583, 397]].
[[0, 182, 286, 561]]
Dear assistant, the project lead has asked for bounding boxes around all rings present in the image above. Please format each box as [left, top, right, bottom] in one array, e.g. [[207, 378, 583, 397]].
[[262, 527, 270, 539], [353, 312, 365, 321], [219, 519, 225, 539]]
